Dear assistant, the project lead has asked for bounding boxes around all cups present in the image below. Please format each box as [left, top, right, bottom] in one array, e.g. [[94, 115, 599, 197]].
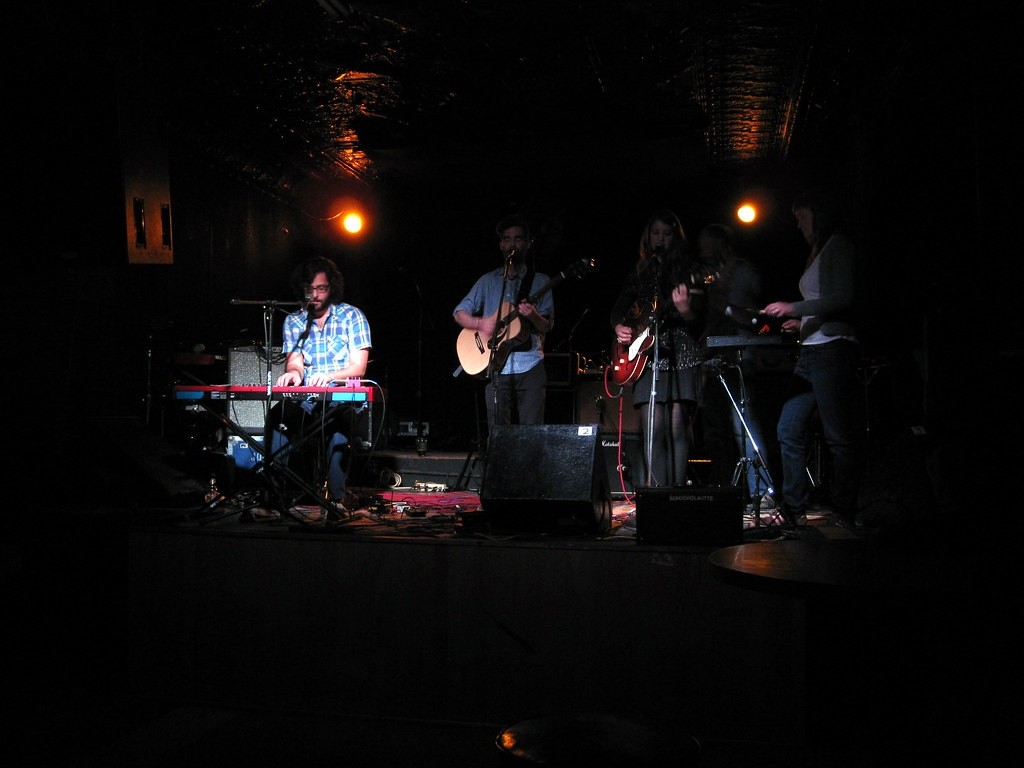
[[417, 437, 427, 455]]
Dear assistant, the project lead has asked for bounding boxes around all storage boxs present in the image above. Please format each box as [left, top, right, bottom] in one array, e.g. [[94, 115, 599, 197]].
[[368, 450, 482, 490]]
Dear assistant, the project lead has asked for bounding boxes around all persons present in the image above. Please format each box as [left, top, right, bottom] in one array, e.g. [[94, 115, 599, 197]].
[[257, 257, 371, 511], [758, 191, 885, 526], [610, 211, 780, 510], [452, 217, 554, 444]]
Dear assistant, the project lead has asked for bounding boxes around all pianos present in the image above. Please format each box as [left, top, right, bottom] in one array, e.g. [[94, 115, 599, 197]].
[[170, 381, 391, 404]]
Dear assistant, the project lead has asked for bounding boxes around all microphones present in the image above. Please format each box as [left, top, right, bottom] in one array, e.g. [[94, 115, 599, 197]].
[[704, 359, 727, 373], [506, 249, 517, 262], [652, 242, 664, 260], [300, 294, 314, 312]]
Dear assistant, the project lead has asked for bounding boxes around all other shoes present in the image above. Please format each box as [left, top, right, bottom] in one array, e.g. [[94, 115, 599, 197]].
[[747, 507, 808, 531], [330, 500, 348, 512], [248, 505, 283, 517], [747, 487, 777, 509], [831, 508, 883, 532]]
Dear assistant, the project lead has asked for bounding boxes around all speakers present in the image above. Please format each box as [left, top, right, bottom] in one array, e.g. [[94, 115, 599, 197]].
[[573, 373, 648, 500], [227, 347, 287, 436], [636, 487, 744, 544], [480, 424, 612, 537]]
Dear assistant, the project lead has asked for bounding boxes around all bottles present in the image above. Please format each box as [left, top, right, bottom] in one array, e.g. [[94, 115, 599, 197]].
[[383, 467, 391, 490]]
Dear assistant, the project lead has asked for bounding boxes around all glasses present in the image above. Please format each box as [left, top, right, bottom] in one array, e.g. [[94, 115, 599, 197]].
[[302, 285, 331, 294]]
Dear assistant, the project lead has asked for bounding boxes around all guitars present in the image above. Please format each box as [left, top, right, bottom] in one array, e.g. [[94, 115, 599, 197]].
[[455, 255, 597, 383], [610, 264, 706, 388]]
